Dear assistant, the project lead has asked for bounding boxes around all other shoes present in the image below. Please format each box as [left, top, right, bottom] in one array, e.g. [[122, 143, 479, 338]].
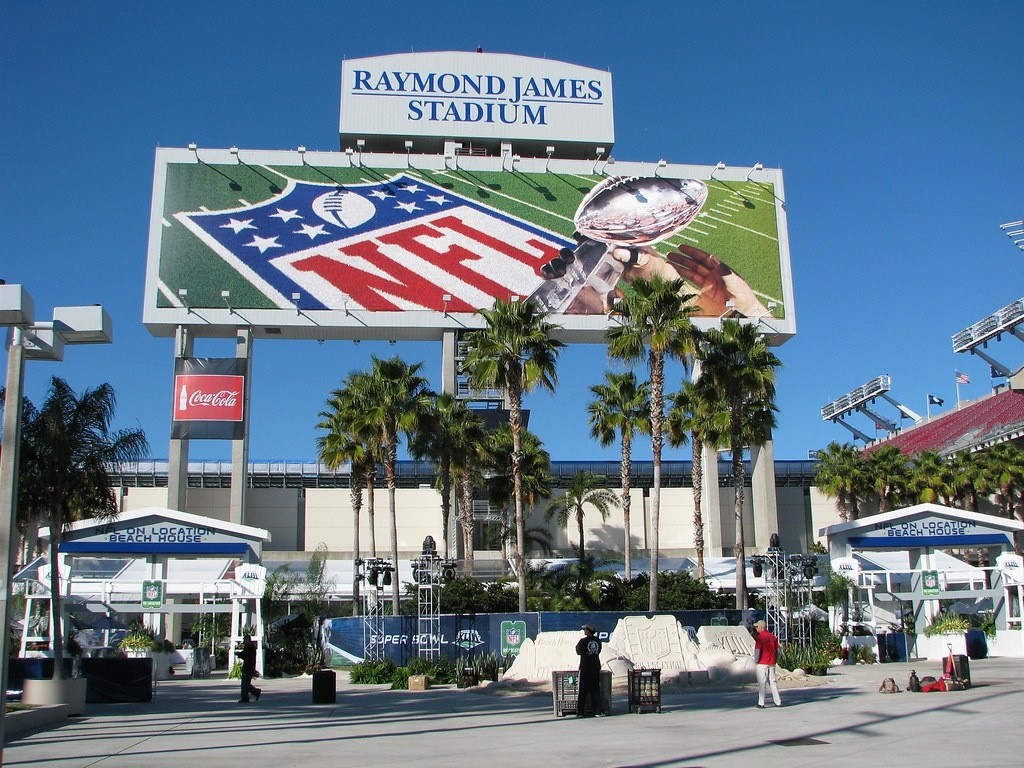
[[255, 689, 261, 701], [237, 698, 249, 703], [757, 705, 764, 708], [775, 705, 782, 707], [577, 714, 584, 718], [595, 711, 606, 718]]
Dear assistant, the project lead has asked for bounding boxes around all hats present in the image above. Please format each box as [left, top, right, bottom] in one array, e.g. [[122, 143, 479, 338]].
[[753, 620, 766, 628], [581, 623, 597, 632], [243, 635, 251, 639]]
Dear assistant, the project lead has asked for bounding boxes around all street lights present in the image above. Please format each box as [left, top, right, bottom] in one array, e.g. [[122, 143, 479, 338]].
[[410, 536, 457, 662], [354, 556, 396, 663], [0, 277, 153, 768], [749, 533, 790, 645], [789, 553, 819, 647]]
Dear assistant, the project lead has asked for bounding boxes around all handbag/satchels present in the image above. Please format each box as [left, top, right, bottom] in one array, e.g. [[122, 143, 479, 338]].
[[252, 669, 260, 678], [921, 676, 937, 685], [943, 678, 966, 691]]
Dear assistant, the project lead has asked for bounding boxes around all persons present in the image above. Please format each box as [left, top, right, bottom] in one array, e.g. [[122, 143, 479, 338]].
[[539, 229, 774, 318], [576, 622, 606, 717], [753, 620, 782, 708], [234, 634, 261, 702]]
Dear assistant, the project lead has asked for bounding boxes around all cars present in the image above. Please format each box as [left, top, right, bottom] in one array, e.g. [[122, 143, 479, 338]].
[[173, 639, 212, 677]]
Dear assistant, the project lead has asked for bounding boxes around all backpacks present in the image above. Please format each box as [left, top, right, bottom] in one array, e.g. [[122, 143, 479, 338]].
[[878, 678, 899, 694]]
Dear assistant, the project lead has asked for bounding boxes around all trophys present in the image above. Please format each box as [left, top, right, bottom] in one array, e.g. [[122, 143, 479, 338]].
[[519, 175, 710, 319]]
